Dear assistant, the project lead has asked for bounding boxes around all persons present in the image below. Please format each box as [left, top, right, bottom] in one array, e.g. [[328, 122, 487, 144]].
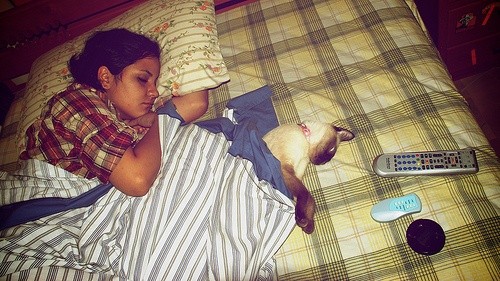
[[4, 26, 209, 281]]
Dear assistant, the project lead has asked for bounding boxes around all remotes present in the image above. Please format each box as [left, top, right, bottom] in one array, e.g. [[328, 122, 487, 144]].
[[370, 192, 422, 223], [373, 150, 479, 178]]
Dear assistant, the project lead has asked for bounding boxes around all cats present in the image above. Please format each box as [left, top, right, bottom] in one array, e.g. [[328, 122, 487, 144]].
[[261, 120, 355, 235]]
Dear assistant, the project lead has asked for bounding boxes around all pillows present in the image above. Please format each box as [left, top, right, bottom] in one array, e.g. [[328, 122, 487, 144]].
[[15, 0, 229, 154]]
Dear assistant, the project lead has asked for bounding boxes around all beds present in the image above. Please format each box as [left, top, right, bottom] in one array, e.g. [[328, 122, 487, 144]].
[[1, 0, 500, 280]]
